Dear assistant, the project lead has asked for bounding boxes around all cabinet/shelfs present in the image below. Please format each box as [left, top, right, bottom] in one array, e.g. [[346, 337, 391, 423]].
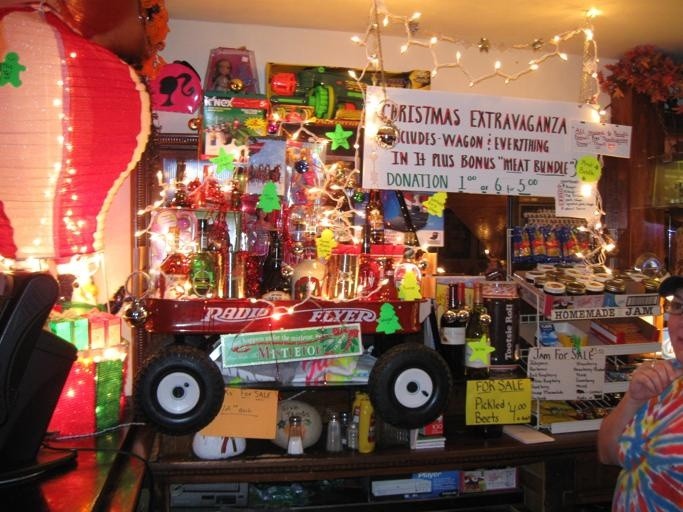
[[504, 225, 668, 437]]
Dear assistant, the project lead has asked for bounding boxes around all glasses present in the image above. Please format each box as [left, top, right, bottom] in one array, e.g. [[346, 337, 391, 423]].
[[662, 299, 682, 315]]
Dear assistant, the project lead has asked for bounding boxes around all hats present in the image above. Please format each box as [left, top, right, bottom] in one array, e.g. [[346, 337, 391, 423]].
[[659, 276, 683, 296]]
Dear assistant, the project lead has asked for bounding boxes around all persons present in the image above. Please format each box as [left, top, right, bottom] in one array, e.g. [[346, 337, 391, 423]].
[[596, 271, 683, 512]]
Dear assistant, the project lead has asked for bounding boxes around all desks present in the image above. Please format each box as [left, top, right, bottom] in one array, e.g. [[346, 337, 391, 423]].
[[149, 422, 631, 512]]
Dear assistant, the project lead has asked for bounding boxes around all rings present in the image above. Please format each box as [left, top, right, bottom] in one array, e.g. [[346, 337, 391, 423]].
[[651, 361, 656, 369]]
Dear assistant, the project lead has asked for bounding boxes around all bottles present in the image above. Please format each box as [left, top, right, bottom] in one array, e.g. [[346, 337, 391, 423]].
[[260, 225, 322, 299], [326, 391, 376, 454], [440, 279, 491, 380], [154, 218, 220, 297], [287, 414, 305, 458], [366, 189, 383, 244]]
